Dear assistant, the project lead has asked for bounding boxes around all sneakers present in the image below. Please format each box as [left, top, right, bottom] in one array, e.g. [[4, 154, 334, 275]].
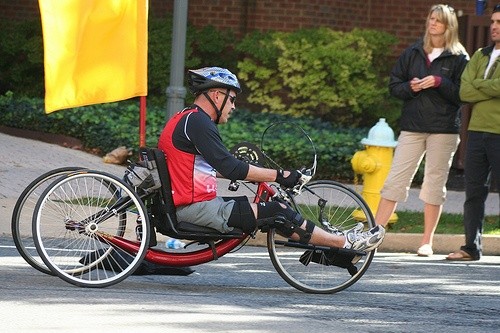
[[343, 223, 364, 236], [347, 225, 385, 252]]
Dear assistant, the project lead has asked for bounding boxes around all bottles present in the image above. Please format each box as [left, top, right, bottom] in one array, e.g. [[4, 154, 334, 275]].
[[165, 238, 185, 249]]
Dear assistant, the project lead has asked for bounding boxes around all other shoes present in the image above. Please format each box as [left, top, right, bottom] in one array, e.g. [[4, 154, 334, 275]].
[[418, 244, 433, 255]]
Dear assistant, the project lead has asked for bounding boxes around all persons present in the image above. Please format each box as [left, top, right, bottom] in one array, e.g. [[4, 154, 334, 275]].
[[374, 5, 470, 255], [159, 67, 386, 265], [448, 5, 500, 260]]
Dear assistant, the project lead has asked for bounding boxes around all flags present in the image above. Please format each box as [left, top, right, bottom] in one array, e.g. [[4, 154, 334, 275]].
[[39, 0, 148, 113]]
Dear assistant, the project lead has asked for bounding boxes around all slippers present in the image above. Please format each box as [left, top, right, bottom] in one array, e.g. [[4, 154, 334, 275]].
[[447, 251, 475, 260]]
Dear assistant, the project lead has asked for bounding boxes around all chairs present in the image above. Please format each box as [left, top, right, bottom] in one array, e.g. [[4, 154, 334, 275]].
[[143, 149, 243, 241]]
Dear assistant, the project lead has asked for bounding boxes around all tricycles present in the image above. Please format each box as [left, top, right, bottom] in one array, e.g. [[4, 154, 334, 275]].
[[10, 119, 377, 295]]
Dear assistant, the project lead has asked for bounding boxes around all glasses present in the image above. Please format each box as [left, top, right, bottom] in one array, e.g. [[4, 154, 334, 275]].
[[210, 90, 236, 104]]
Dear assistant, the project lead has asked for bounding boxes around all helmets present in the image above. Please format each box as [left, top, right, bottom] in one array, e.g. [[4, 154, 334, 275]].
[[188, 66, 242, 95]]
